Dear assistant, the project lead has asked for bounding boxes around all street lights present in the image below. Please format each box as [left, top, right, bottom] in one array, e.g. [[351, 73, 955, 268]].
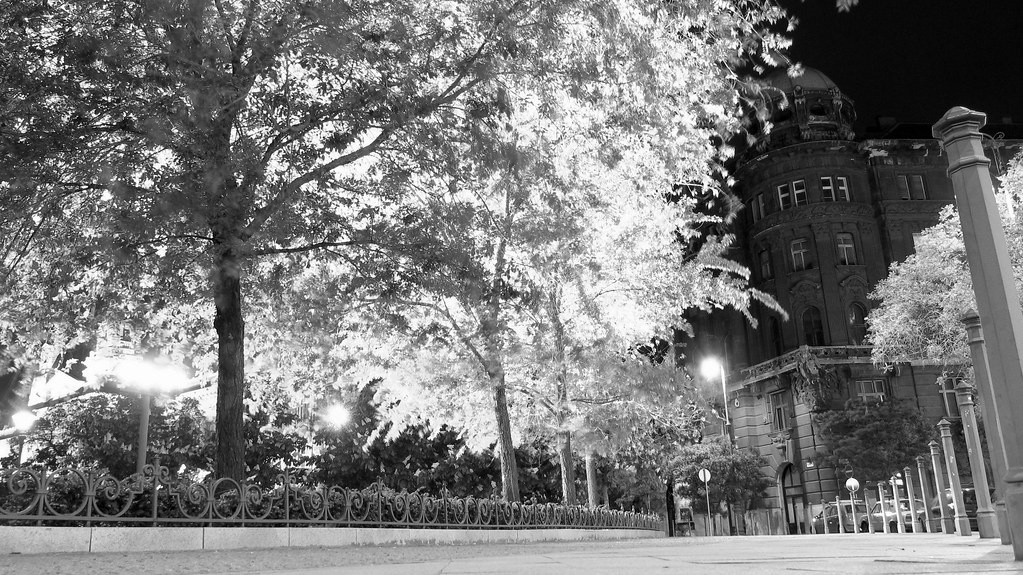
[[702, 358, 731, 427]]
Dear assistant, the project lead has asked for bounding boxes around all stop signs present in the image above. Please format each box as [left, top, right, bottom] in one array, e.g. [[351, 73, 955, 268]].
[[844, 464, 854, 479]]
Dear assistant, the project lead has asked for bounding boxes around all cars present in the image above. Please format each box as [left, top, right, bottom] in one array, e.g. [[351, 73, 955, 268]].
[[857, 498, 930, 533], [918, 487, 996, 534], [809, 499, 874, 534]]
